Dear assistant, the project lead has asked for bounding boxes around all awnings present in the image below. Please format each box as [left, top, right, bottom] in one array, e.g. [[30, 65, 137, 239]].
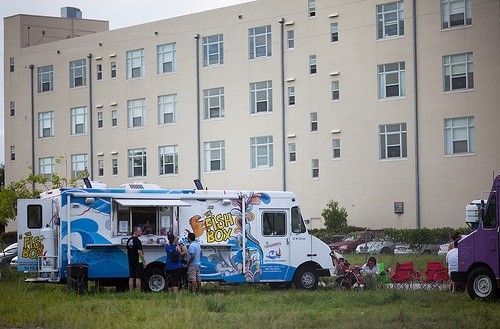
[[112, 198, 192, 207]]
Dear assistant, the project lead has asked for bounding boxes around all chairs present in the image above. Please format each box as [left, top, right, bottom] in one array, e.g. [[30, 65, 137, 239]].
[[417, 261, 450, 292], [389, 262, 424, 292]]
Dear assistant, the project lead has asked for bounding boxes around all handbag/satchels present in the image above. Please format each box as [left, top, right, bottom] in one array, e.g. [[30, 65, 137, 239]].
[[170, 251, 180, 263]]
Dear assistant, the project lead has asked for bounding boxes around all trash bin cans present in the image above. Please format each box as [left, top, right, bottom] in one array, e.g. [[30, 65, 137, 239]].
[[65, 262, 90, 297]]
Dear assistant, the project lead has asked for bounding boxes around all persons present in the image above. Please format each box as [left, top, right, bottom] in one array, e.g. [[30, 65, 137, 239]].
[[125, 226, 147, 292], [446, 228, 474, 293], [334, 257, 379, 291], [165, 234, 181, 293], [187, 233, 203, 296], [174, 237, 189, 290], [140, 220, 152, 234]]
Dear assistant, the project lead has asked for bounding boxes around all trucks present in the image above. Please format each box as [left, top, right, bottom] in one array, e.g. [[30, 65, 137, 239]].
[[446, 172, 500, 302], [16, 180, 344, 291]]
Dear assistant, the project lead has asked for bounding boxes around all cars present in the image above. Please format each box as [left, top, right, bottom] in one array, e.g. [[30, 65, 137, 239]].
[[393, 237, 448, 256], [329, 229, 382, 254], [355, 233, 403, 255], [0, 242, 18, 270]]
